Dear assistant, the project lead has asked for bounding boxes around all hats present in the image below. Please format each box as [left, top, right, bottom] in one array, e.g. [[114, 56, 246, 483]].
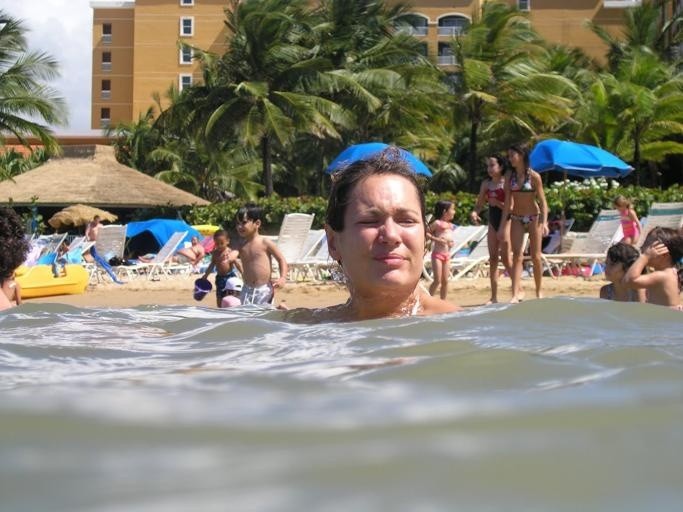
[[221, 278, 243, 291]]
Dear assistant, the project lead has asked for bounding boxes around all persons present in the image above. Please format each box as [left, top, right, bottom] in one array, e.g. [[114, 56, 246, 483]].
[[57, 238, 70, 257], [214, 202, 289, 310], [0, 205, 34, 314], [618, 225, 682, 311], [468, 153, 526, 306], [298, 142, 467, 321], [599, 242, 647, 303], [424, 199, 456, 300], [84, 215, 104, 241], [611, 194, 641, 248], [495, 141, 549, 305], [527, 221, 570, 255], [1, 271, 23, 306], [136, 235, 205, 266], [200, 228, 243, 309]]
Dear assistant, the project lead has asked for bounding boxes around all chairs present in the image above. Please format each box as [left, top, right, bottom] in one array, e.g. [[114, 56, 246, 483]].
[[17, 212, 314, 283], [304, 201, 683, 281]]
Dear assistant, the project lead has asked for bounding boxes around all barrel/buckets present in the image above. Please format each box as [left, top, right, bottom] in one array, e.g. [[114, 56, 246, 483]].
[[191, 278, 212, 302]]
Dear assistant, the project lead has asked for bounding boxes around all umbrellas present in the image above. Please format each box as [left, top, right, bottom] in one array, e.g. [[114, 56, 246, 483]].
[[45, 204, 118, 228], [526, 137, 635, 184], [325, 141, 435, 179]]
